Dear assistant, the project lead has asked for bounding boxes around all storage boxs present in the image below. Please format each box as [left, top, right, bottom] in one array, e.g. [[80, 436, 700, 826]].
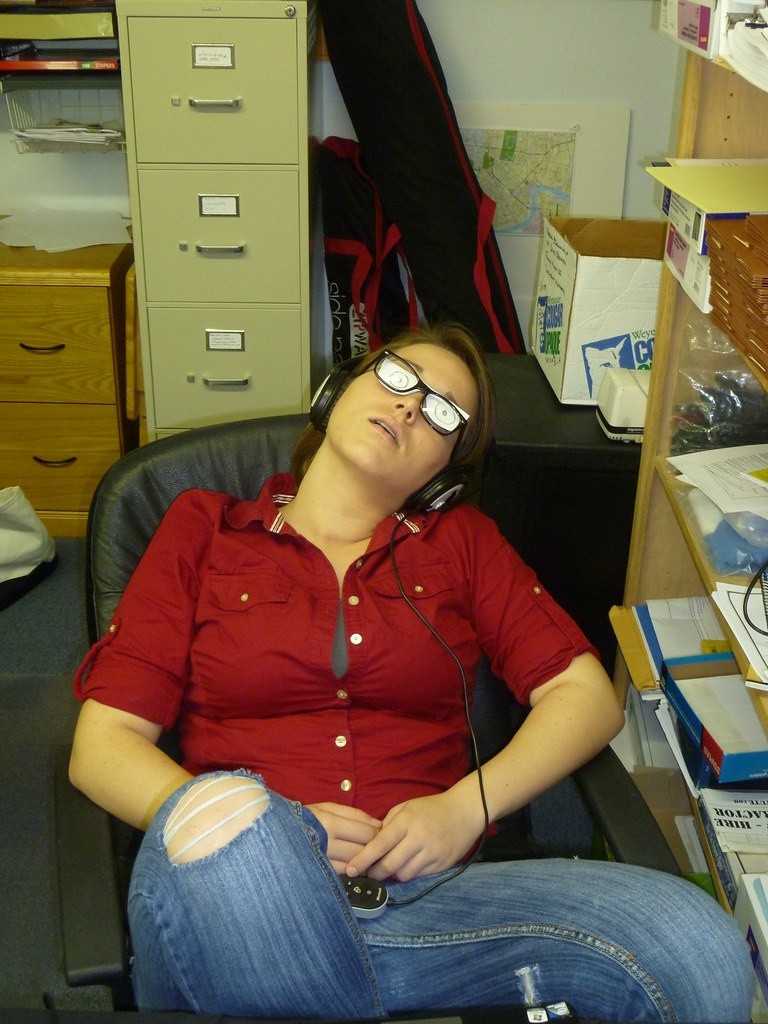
[[530, 212, 668, 410]]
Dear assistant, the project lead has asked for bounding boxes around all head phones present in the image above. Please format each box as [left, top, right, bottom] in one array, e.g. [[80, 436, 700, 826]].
[[307, 355, 473, 514]]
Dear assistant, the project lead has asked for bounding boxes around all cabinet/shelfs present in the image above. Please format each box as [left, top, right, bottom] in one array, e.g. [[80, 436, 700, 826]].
[[0, 215, 136, 539], [114, 0, 312, 448]]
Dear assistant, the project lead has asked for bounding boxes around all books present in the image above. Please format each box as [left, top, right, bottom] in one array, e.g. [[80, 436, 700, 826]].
[[718, 1, 768, 93]]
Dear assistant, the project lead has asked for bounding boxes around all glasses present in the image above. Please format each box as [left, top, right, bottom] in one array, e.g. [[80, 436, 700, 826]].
[[358, 348, 470, 469]]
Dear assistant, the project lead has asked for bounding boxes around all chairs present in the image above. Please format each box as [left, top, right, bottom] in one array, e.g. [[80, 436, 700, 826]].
[[50, 411, 690, 1024]]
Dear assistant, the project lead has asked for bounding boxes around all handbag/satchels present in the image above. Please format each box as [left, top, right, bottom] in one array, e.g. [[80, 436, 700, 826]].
[[323, 134, 418, 368], [320, 1, 525, 357]]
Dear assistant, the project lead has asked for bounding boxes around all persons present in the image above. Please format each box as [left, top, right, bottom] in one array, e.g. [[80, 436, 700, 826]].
[[67, 330, 757, 1024]]
